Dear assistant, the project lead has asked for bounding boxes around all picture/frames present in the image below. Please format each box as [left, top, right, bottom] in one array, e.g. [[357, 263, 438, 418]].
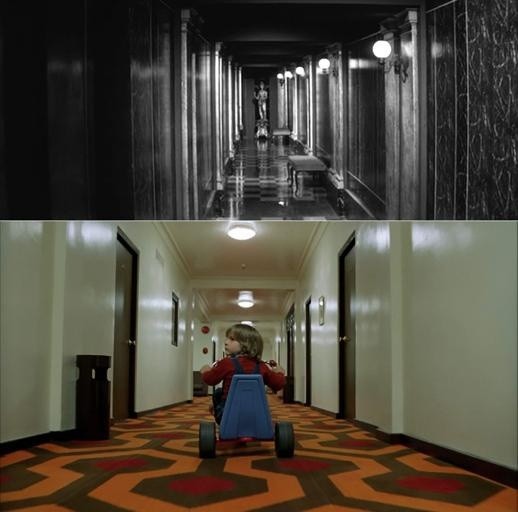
[[319, 295, 324, 326]]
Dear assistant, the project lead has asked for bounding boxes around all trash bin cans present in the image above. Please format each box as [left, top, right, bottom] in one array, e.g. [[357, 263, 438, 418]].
[[283, 376, 294, 404], [75, 354, 110, 440]]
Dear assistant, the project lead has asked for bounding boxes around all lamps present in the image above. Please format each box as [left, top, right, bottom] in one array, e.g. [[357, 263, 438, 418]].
[[234, 288, 257, 309], [370, 40, 409, 84], [275, 64, 308, 88], [318, 56, 337, 78], [226, 222, 257, 241]]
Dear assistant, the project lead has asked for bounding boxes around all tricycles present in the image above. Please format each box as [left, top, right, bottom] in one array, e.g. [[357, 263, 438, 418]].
[[196, 357, 296, 458]]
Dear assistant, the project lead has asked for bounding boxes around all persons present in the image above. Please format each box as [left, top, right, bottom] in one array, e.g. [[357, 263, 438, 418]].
[[254, 80, 268, 120], [200, 324, 285, 426]]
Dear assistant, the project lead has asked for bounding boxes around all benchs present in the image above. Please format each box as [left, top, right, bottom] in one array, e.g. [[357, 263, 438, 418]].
[[285, 154, 330, 197], [270, 128, 291, 146]]
